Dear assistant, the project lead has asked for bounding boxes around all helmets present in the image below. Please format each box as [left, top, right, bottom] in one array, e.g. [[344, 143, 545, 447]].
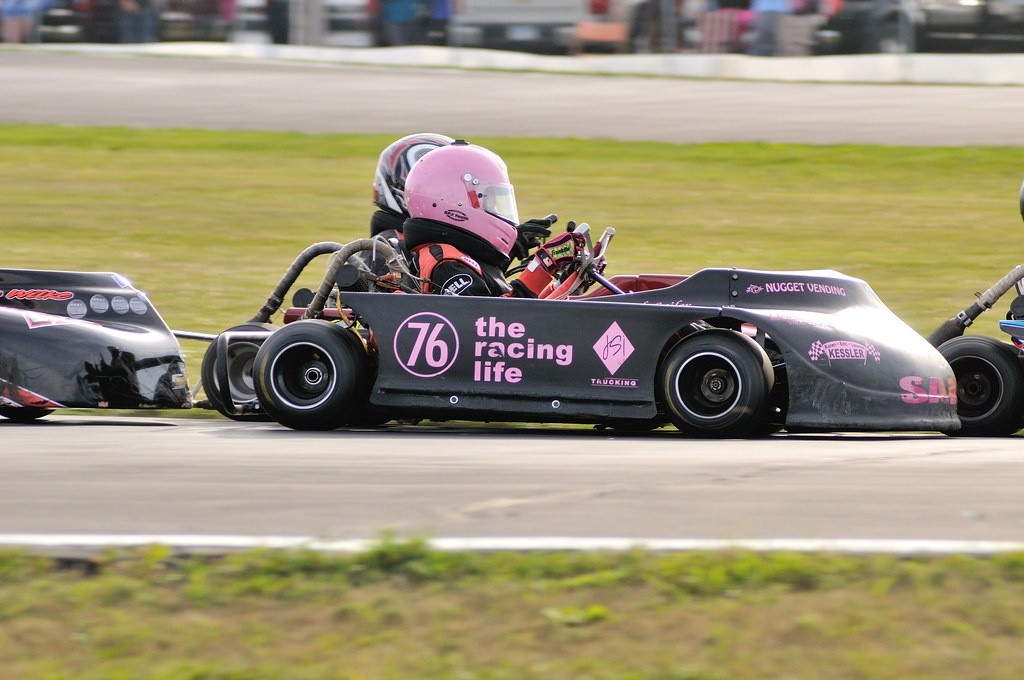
[[372, 132, 456, 220], [403, 139, 519, 268]]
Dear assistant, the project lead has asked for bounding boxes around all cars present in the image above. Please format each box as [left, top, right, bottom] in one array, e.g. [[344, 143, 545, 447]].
[[1, 1, 1024, 59]]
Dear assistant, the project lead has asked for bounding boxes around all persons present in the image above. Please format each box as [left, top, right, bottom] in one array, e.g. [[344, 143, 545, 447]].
[[717, 0, 753, 42], [0, 0, 164, 43], [404, 140, 605, 304], [368, 1, 450, 46], [358, 134, 552, 292]]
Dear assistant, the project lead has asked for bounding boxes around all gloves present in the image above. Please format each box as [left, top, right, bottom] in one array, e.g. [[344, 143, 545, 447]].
[[514, 214, 558, 259], [517, 232, 607, 297]]
